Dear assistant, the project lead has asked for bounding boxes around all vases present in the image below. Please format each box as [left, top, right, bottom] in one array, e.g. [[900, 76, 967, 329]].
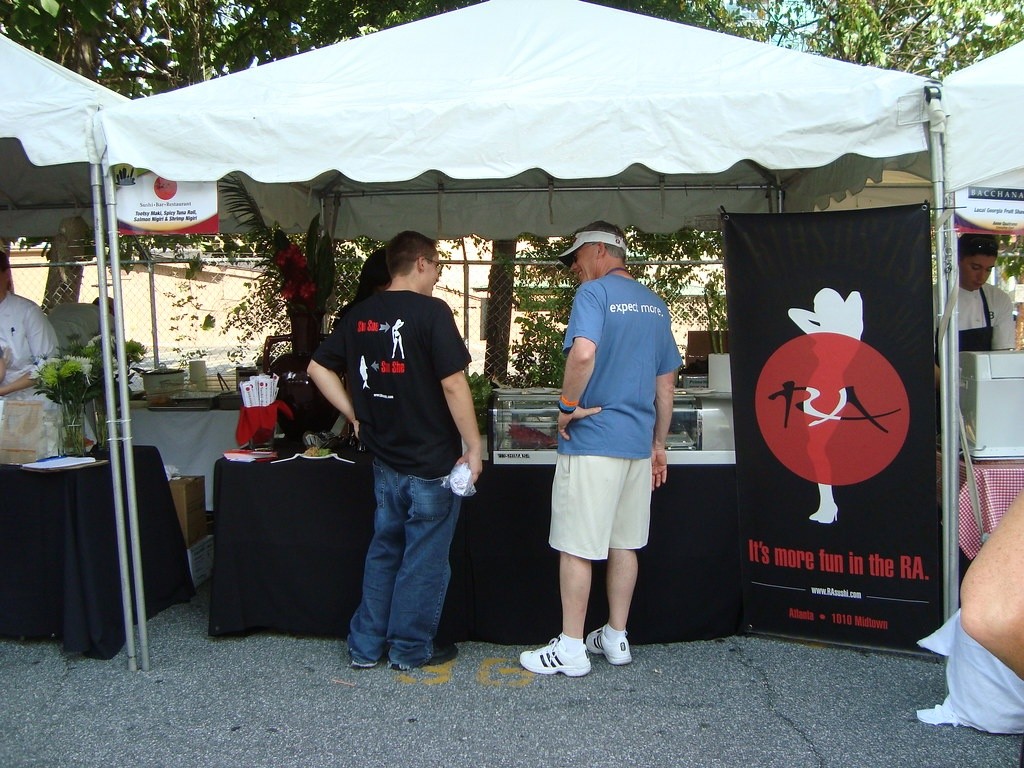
[[93, 397, 121, 452], [56, 403, 87, 458]]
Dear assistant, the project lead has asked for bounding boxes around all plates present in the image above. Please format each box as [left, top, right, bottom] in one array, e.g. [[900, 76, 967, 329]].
[[525, 388, 557, 393], [172, 391, 220, 399], [299, 452, 336, 460], [685, 388, 715, 393], [492, 388, 522, 395]]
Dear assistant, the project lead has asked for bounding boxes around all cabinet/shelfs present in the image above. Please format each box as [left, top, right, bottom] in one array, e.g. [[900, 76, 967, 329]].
[[488, 388, 736, 467]]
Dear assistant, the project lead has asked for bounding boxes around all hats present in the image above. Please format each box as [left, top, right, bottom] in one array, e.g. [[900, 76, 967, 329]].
[[557, 231, 627, 267]]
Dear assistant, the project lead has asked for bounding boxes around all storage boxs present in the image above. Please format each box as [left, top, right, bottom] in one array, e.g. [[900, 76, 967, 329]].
[[187, 535, 214, 588], [168, 476, 207, 549]]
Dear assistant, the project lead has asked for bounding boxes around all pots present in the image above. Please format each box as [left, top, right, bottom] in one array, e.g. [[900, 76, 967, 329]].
[[141, 362, 185, 392]]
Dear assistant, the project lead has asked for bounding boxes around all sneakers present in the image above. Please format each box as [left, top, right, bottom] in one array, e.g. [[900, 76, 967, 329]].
[[520, 634, 591, 676], [392, 637, 458, 670], [586, 624, 632, 665], [351, 644, 390, 668]]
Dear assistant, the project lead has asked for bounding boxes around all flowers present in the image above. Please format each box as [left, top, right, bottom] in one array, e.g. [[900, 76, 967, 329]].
[[65, 328, 148, 447], [27, 344, 105, 455]]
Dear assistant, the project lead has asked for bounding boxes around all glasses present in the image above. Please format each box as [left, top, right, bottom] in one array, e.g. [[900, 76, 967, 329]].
[[573, 243, 608, 263], [417, 256, 444, 275], [967, 238, 999, 250]]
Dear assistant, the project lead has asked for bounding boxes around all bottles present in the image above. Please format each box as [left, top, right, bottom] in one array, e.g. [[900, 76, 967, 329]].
[[189, 361, 206, 391]]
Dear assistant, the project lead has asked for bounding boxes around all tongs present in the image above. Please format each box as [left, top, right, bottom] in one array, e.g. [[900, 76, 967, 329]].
[[217, 373, 230, 392]]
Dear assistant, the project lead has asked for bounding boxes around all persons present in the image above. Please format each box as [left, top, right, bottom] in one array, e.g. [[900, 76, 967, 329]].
[[960, 487, 1024, 683], [307, 230, 483, 672], [48, 296, 116, 358], [935, 233, 1016, 436], [518, 221, 681, 676], [332, 247, 392, 329], [0, 251, 98, 455]]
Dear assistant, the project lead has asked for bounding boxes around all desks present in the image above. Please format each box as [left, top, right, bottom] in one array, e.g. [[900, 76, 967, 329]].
[[0, 444, 195, 661], [208, 447, 744, 645], [936, 447, 1024, 587]]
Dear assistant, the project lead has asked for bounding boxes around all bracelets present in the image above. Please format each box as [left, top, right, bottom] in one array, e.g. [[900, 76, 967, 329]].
[[559, 400, 575, 411], [557, 404, 576, 414], [560, 395, 579, 406]]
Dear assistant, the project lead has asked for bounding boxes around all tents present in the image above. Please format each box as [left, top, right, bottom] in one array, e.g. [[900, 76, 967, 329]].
[[93, 0, 952, 671], [0, 32, 261, 676], [941, 38, 1024, 620]]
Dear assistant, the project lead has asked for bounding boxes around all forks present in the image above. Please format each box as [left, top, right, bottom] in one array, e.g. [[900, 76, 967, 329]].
[[329, 453, 356, 464], [270, 453, 303, 463]]
